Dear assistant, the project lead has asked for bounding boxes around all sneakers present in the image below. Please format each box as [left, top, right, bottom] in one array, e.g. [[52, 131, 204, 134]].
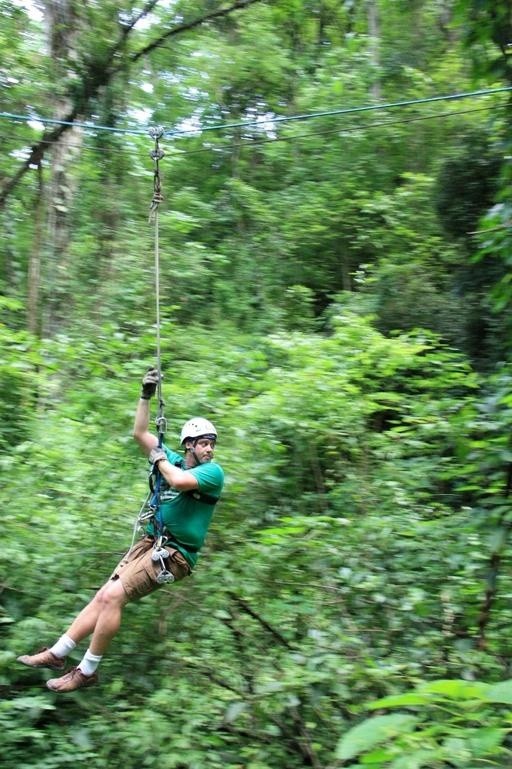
[[16, 649, 66, 671], [46, 667, 100, 693]]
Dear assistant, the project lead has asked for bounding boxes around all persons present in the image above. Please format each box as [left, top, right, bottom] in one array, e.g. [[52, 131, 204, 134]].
[[15, 365, 225, 693]]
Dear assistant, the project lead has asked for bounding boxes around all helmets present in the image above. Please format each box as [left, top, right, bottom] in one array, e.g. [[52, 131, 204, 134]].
[[179, 417, 218, 446]]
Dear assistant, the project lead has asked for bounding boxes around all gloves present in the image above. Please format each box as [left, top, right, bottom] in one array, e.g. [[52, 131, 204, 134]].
[[140, 367, 163, 400], [148, 447, 168, 466]]
